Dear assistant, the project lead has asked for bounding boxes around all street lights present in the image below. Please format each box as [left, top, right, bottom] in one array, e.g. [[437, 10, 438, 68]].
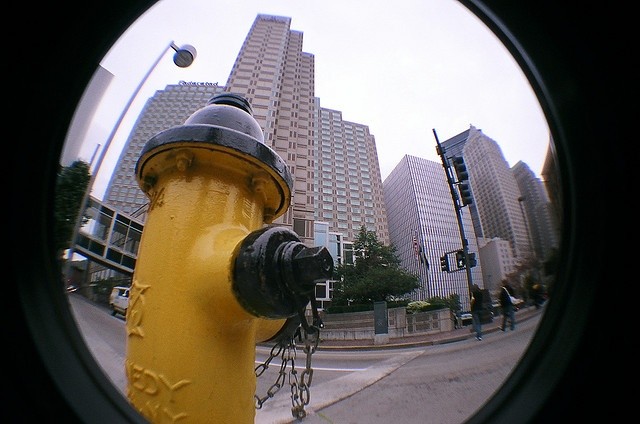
[[518, 196, 534, 253], [64, 40, 196, 293]]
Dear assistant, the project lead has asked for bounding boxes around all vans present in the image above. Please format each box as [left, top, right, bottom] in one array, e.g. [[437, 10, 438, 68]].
[[108, 287, 131, 316]]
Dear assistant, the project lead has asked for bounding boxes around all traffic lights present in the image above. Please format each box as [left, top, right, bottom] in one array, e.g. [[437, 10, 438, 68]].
[[452, 156, 468, 180], [440, 253, 449, 273], [456, 251, 466, 268], [468, 253, 476, 268], [459, 184, 472, 204]]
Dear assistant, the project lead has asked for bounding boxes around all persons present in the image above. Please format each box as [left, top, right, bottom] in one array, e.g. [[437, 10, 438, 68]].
[[496, 278, 516, 331], [469, 283, 484, 341]]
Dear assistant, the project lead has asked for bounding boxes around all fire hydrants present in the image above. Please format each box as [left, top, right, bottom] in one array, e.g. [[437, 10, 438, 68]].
[[126, 93, 333, 424]]
